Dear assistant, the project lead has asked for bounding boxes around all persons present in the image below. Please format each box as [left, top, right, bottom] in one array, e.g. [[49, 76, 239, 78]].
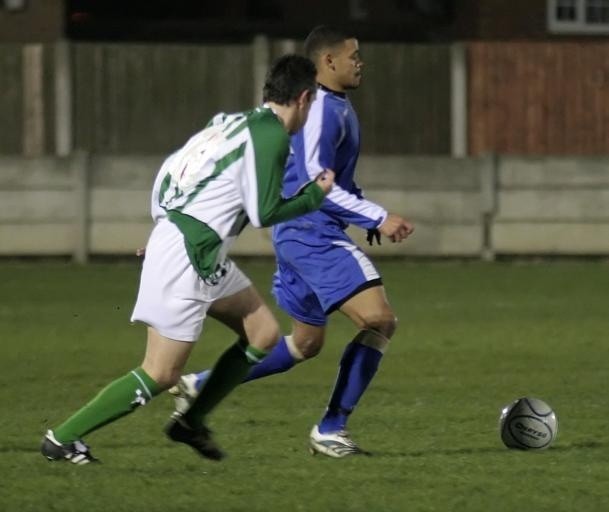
[[40, 53, 336, 469], [167, 21, 416, 460]]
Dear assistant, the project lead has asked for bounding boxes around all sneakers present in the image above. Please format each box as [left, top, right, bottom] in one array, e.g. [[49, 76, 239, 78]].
[[164, 374, 223, 461], [309, 424, 371, 459], [41, 429, 103, 465]]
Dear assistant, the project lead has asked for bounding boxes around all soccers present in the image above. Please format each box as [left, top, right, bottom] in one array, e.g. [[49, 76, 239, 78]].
[[501, 397, 557, 450]]
